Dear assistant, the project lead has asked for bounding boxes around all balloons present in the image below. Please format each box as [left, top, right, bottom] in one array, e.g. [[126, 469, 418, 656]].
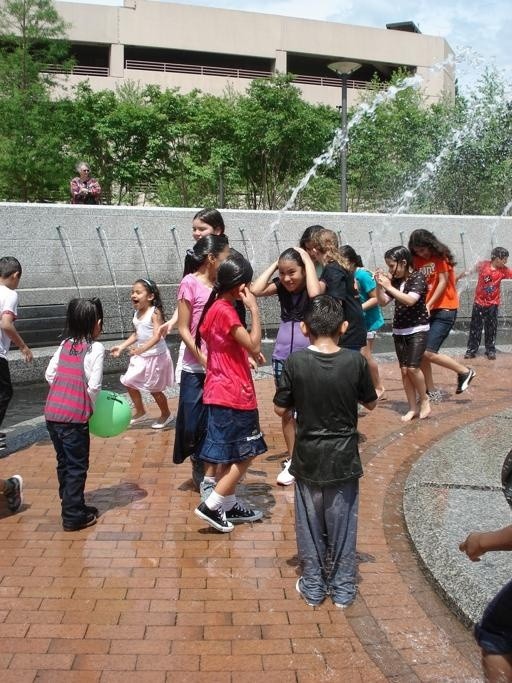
[[88, 389, 134, 439]]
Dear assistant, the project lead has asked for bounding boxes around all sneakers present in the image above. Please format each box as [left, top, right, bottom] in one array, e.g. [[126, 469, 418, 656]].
[[456, 366, 476, 394], [4, 474, 23, 511], [276, 459, 295, 485], [295, 576, 321, 607], [192, 470, 263, 532], [487, 350, 496, 360], [464, 348, 477, 358]]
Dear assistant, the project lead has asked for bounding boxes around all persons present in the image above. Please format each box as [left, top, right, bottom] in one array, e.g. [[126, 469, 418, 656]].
[[41, 293, 102, 533], [0, 253, 37, 450], [104, 274, 177, 430], [70, 159, 104, 205], [0, 471, 25, 517], [455, 443, 511, 682], [250, 243, 327, 489], [154, 202, 385, 403], [373, 229, 476, 421], [455, 243, 512, 362], [192, 249, 267, 536], [171, 231, 264, 503], [271, 289, 380, 608]]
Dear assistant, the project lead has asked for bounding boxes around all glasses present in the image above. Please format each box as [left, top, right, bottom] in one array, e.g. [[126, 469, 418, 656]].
[[84, 170, 89, 172]]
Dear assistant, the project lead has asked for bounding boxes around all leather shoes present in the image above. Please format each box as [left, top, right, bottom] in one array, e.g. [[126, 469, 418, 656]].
[[63, 514, 96, 531], [130, 411, 148, 424], [86, 506, 98, 515], [151, 412, 174, 429]]
